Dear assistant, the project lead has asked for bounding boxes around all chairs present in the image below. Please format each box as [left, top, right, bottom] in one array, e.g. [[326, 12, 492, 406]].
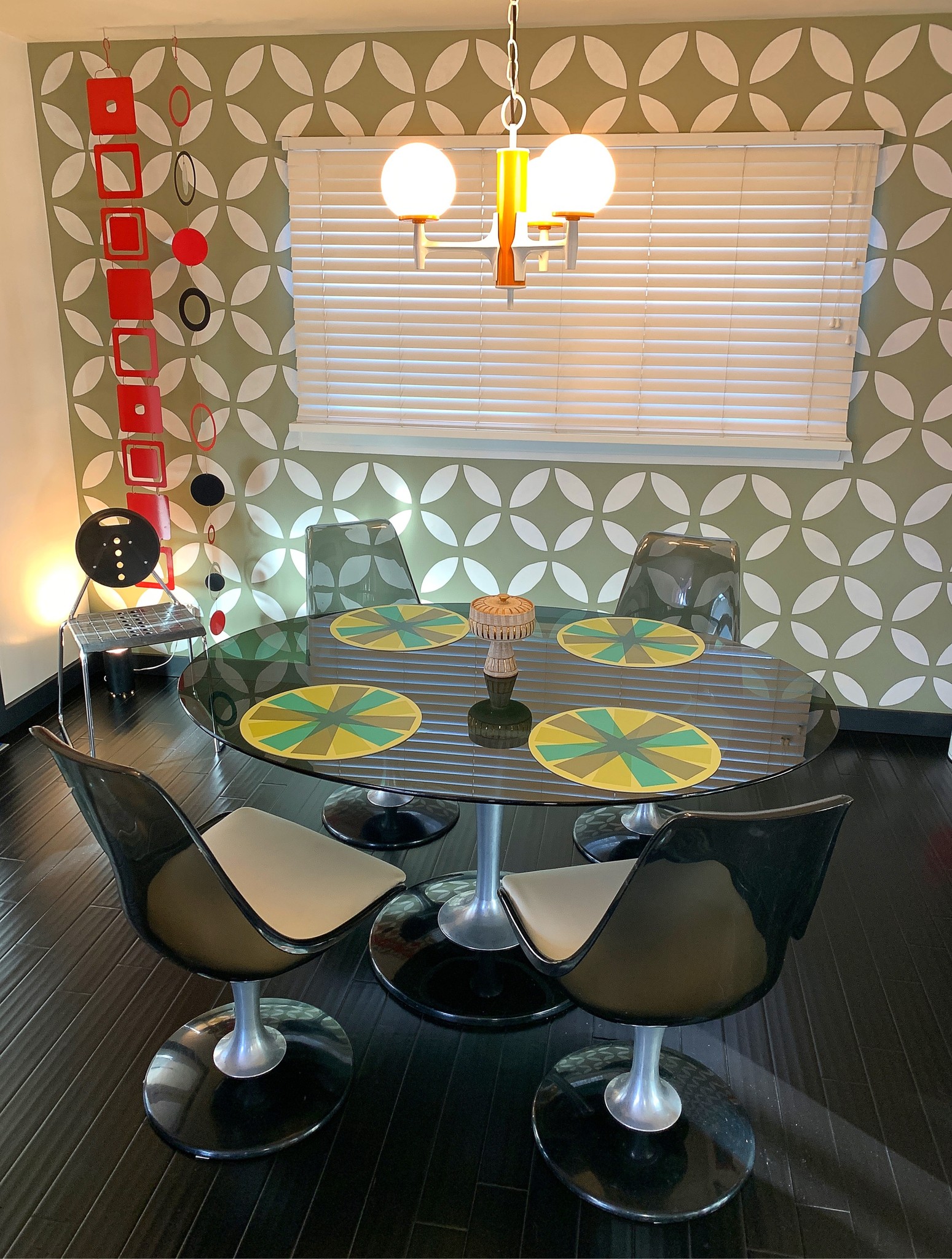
[[499, 795, 855, 1226], [59, 507, 224, 758], [29, 724, 407, 1163], [308, 516, 461, 850], [571, 532, 741, 862]]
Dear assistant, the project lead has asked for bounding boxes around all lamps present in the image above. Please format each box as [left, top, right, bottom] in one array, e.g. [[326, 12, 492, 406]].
[[380, 0, 618, 312], [103, 648, 135, 698]]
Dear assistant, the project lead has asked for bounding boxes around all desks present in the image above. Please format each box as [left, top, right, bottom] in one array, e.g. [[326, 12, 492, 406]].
[[178, 602, 840, 1032]]
